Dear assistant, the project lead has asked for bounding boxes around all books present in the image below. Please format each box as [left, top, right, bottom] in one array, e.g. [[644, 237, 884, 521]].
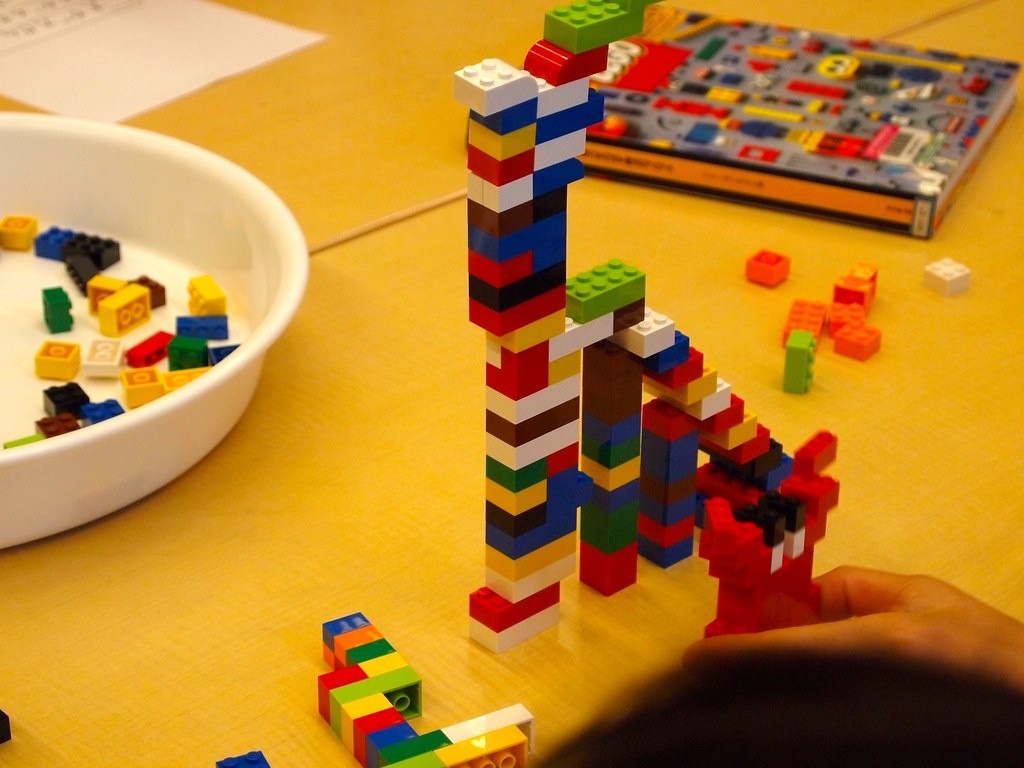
[[575, 4, 1022, 239]]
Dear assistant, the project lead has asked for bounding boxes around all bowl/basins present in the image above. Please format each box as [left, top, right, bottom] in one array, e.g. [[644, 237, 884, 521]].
[[0, 111, 308, 552]]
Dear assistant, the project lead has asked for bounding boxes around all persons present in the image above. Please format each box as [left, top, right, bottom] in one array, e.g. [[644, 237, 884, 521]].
[[534, 566, 1024, 768]]
